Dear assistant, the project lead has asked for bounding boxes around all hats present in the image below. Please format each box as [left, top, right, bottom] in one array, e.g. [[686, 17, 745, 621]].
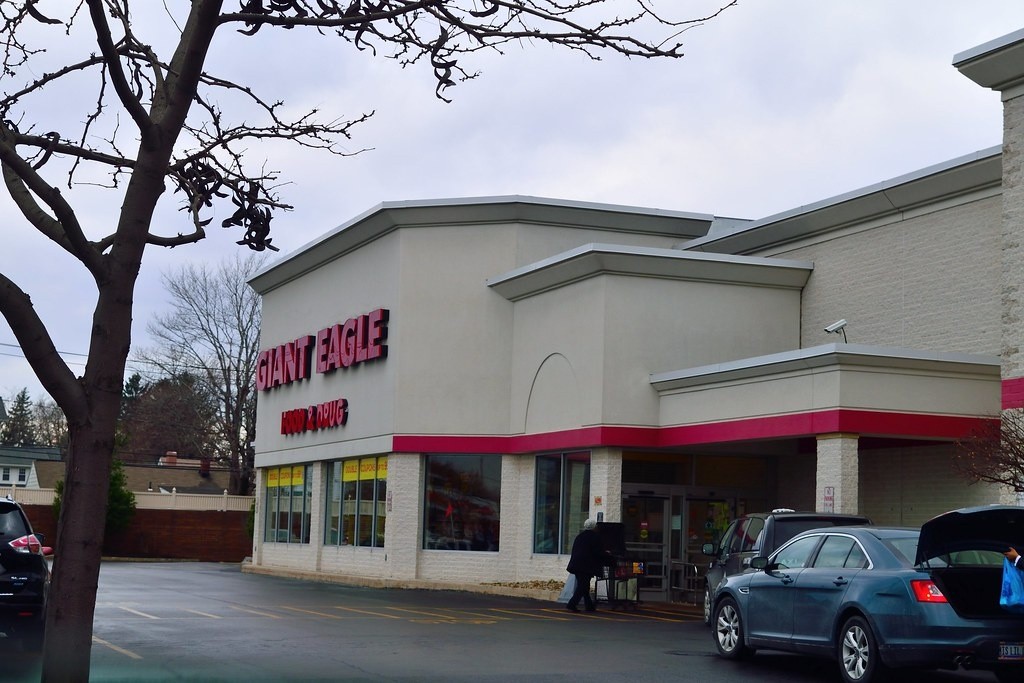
[[584, 519, 597, 531]]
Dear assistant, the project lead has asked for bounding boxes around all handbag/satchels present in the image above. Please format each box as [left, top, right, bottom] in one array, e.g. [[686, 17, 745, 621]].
[[999, 556, 1024, 614], [557, 573, 576, 603]]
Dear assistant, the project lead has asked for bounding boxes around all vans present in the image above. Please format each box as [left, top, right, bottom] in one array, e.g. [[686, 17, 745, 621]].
[[701, 508, 876, 628]]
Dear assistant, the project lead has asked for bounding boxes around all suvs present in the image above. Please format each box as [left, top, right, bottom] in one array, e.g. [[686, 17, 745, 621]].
[[0, 493, 55, 649]]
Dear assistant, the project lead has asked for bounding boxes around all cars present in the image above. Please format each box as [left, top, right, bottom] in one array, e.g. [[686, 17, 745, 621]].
[[711, 502, 1024, 683]]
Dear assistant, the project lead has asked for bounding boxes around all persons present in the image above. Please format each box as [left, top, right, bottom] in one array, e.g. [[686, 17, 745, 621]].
[[565, 519, 612, 612], [1004, 547, 1024, 573]]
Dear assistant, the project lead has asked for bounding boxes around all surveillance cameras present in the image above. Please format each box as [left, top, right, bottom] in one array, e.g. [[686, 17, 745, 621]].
[[824, 319, 847, 333]]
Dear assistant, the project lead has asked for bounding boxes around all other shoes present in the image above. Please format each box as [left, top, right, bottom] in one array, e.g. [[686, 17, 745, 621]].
[[586, 607, 596, 613], [566, 602, 581, 613]]
